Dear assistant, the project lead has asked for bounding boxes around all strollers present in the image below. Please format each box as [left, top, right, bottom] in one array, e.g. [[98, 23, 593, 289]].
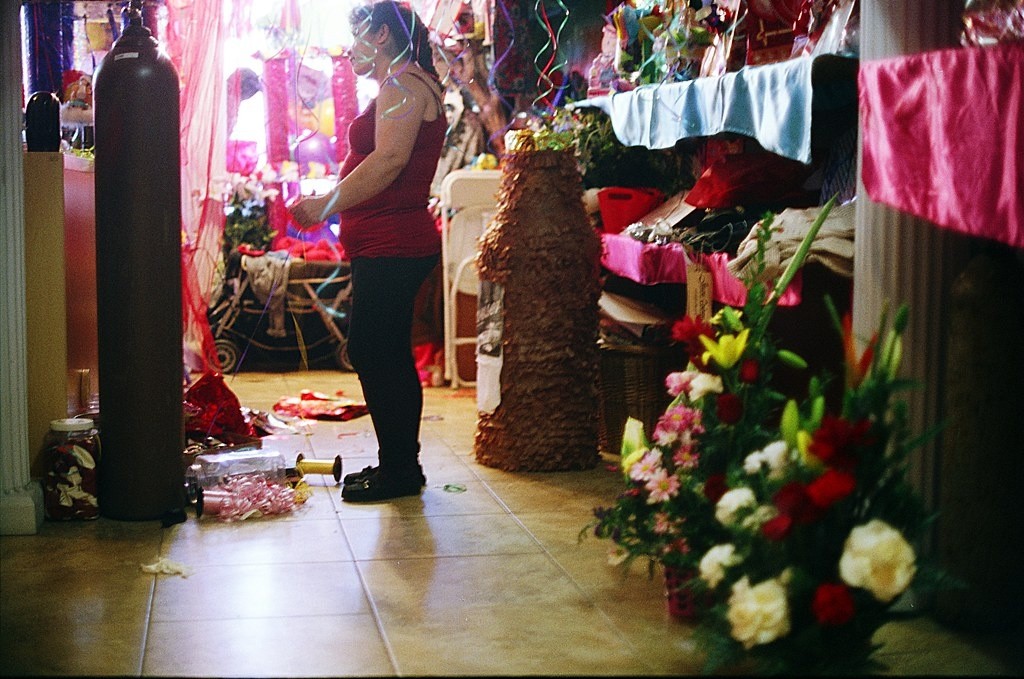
[[195, 248, 356, 374]]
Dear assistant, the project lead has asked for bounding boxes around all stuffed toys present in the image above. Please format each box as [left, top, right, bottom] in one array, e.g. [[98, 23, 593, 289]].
[[61, 75, 93, 159]]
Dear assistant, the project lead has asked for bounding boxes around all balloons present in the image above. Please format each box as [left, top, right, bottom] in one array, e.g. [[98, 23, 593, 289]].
[[293, 134, 334, 175]]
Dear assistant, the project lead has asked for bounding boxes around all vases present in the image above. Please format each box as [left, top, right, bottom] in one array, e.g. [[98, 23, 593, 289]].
[[662, 565, 721, 624]]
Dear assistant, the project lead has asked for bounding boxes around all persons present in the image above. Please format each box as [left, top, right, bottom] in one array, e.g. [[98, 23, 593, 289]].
[[289, 0, 448, 503]]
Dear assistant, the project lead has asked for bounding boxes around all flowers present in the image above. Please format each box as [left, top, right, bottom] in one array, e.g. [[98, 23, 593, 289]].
[[691, 300, 939, 677], [576, 193, 845, 586]]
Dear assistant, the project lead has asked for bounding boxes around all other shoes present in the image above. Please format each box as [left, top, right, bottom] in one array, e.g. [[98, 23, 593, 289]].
[[342, 465, 425, 502]]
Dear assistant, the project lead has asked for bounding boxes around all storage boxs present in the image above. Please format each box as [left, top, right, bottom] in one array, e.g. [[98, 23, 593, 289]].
[[596, 340, 688, 474]]
